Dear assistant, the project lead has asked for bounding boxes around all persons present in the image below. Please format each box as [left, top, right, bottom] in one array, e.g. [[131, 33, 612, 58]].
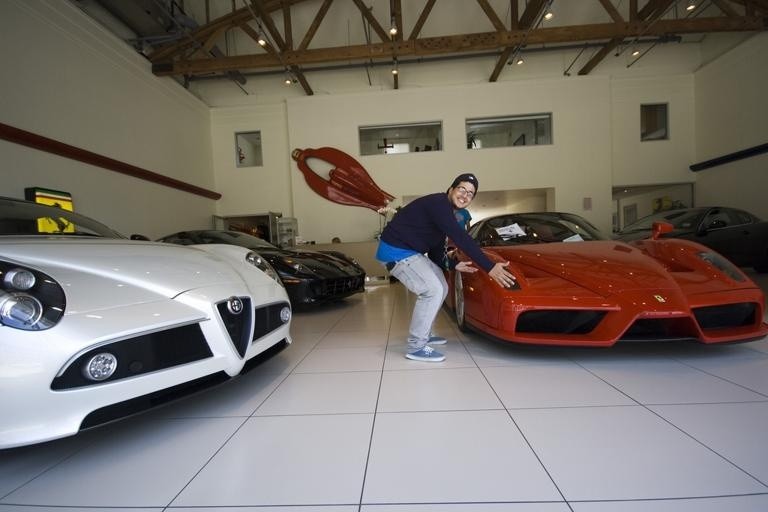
[[376, 173, 515, 361], [332, 238, 341, 243]]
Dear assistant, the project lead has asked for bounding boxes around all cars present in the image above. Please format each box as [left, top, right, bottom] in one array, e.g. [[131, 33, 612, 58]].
[[611, 205, 768, 274]]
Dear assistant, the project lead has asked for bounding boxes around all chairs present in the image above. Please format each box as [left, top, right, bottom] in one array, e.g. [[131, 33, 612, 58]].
[[543, 224, 569, 241]]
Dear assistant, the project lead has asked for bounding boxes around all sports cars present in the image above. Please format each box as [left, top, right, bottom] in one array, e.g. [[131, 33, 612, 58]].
[[442, 210, 768, 347], [154, 229, 367, 307], [0, 196, 294, 450]]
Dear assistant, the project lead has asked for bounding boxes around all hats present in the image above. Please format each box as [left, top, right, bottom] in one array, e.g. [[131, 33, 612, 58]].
[[454, 174, 478, 189]]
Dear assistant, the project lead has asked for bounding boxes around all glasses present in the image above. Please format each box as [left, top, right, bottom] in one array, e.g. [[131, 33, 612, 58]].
[[457, 187, 474, 198]]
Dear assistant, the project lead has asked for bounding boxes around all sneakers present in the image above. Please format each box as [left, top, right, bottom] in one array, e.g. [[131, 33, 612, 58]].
[[406, 337, 447, 361]]
